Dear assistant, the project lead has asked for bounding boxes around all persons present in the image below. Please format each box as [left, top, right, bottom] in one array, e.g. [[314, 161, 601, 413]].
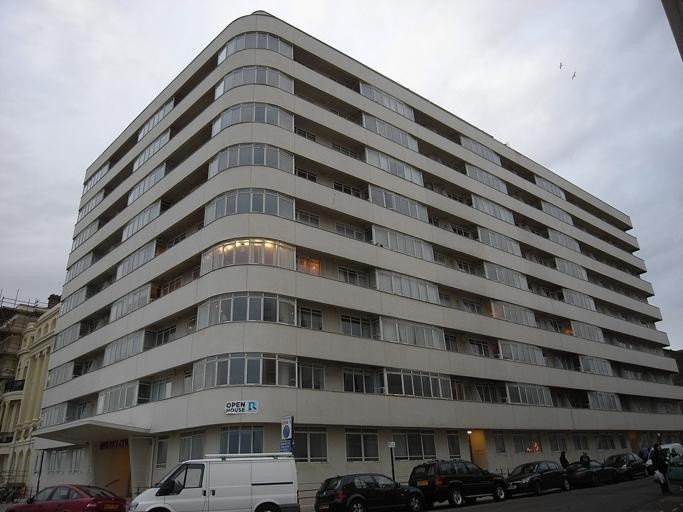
[[559, 450, 569, 467], [579, 451, 590, 462], [650, 443, 673, 495]]
